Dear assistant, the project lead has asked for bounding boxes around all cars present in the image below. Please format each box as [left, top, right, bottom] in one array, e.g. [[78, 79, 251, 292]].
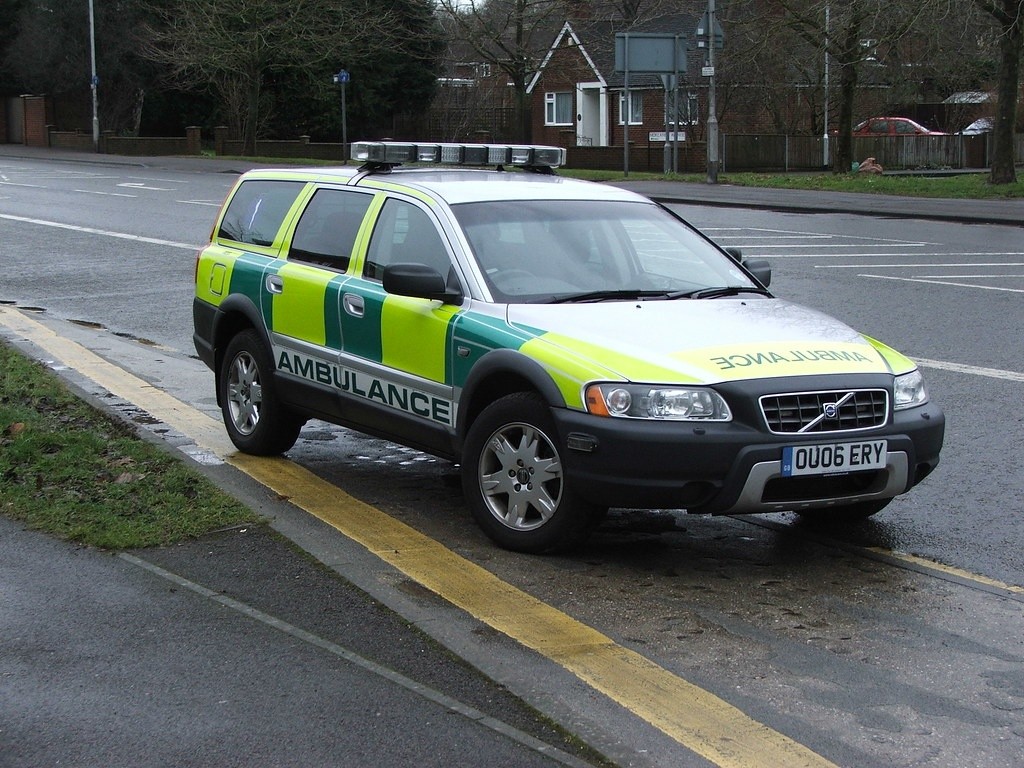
[[831, 116, 949, 137], [953, 116, 996, 135], [191, 138, 947, 559]]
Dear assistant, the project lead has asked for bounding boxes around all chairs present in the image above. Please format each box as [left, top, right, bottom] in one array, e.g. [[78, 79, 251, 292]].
[[302, 212, 378, 258], [531, 224, 605, 289]]
